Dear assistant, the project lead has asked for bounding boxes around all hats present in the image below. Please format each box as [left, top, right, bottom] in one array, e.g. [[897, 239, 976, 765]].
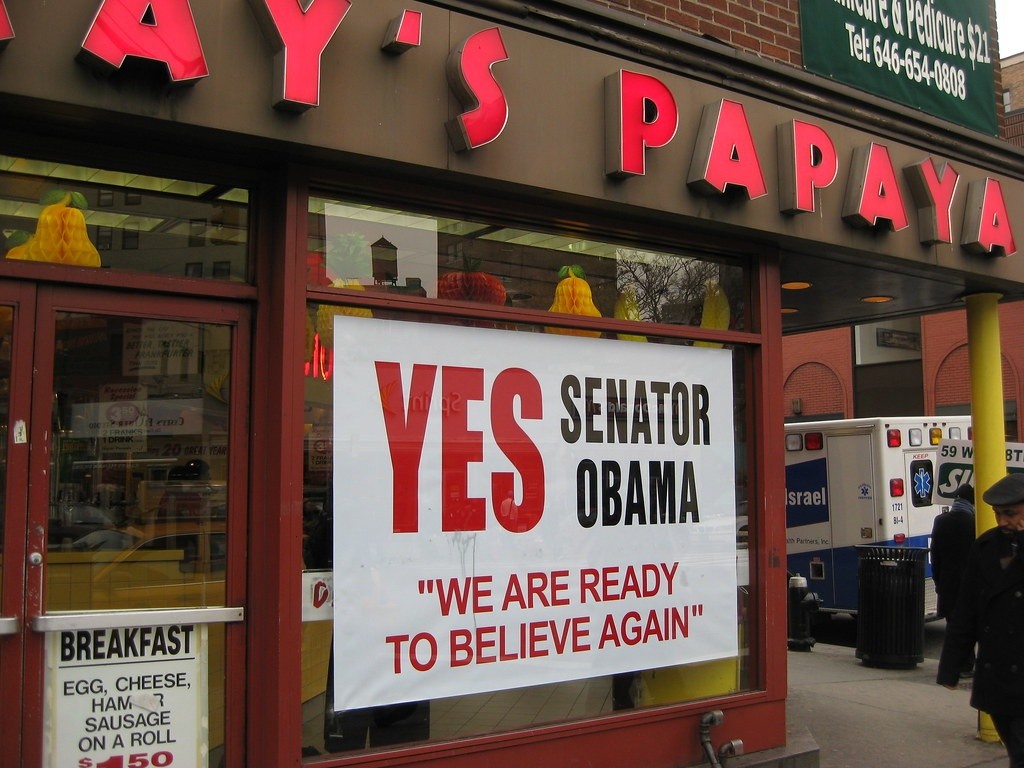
[[982, 473, 1024, 506]]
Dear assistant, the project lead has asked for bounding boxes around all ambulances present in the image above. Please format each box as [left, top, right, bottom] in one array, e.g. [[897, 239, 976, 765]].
[[784, 415, 975, 622]]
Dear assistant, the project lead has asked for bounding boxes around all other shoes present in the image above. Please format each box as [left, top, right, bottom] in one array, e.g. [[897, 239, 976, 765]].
[[961, 671, 975, 678]]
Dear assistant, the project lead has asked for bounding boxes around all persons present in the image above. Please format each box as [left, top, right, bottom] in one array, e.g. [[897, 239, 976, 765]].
[[152, 459, 219, 561], [302, 499, 323, 533], [930, 484, 976, 679], [936, 473, 1024, 768]]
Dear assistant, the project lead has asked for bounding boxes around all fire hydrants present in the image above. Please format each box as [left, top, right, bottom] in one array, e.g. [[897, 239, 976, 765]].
[[787, 572, 823, 652]]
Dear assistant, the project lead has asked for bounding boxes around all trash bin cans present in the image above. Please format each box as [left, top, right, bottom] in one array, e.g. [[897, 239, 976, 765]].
[[849, 543, 930, 670]]
